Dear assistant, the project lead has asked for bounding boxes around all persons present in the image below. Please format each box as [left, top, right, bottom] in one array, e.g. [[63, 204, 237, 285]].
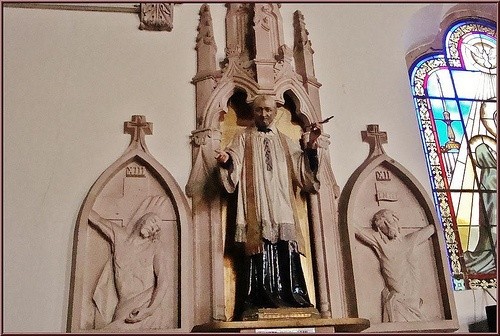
[[88, 207, 169, 325], [352, 208, 437, 323], [215, 94, 323, 319]]
